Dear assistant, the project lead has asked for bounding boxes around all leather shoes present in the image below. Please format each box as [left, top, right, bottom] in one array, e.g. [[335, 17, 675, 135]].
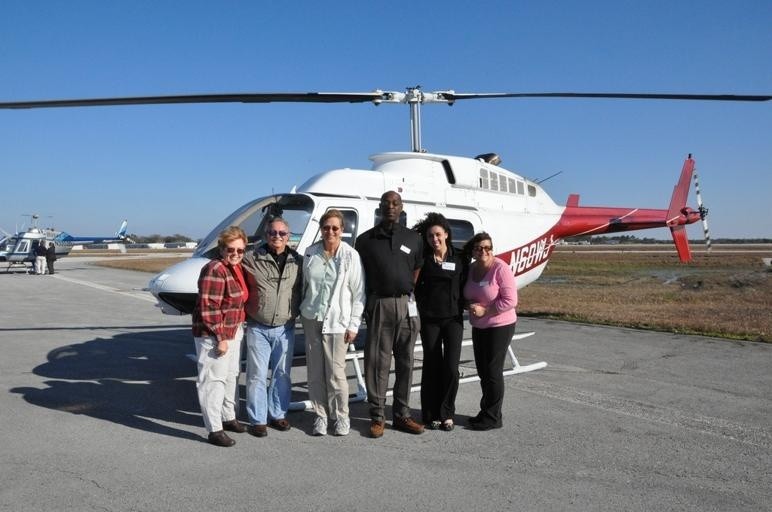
[[443, 419, 455, 432], [465, 413, 488, 424], [368, 420, 386, 437], [271, 419, 290, 432], [207, 431, 235, 447], [251, 425, 268, 437], [393, 418, 425, 434], [221, 419, 249, 434], [469, 419, 498, 431], [432, 421, 442, 430]]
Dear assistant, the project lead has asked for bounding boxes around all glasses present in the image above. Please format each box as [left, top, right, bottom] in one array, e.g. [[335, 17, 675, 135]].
[[222, 247, 248, 254], [473, 247, 493, 251], [267, 230, 291, 236], [321, 225, 342, 231]]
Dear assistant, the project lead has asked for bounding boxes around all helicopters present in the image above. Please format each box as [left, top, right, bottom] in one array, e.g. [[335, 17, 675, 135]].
[[0, 85, 771, 414], [0, 211, 137, 276]]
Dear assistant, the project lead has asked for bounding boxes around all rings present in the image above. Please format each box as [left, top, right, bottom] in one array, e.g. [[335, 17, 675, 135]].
[[473, 311, 475, 316]]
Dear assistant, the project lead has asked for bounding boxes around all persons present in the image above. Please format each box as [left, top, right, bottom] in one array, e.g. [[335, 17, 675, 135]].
[[296, 207, 368, 437], [460, 232, 518, 431], [238, 216, 305, 437], [45, 242, 57, 275], [35, 240, 48, 275], [415, 212, 469, 431], [192, 225, 250, 448], [354, 190, 426, 439]]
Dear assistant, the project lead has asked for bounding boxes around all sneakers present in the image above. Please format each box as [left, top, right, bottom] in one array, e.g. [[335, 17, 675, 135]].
[[312, 415, 328, 436], [334, 417, 350, 437]]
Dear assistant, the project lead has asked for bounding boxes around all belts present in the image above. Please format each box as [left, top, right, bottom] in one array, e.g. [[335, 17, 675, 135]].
[[378, 290, 412, 300]]
